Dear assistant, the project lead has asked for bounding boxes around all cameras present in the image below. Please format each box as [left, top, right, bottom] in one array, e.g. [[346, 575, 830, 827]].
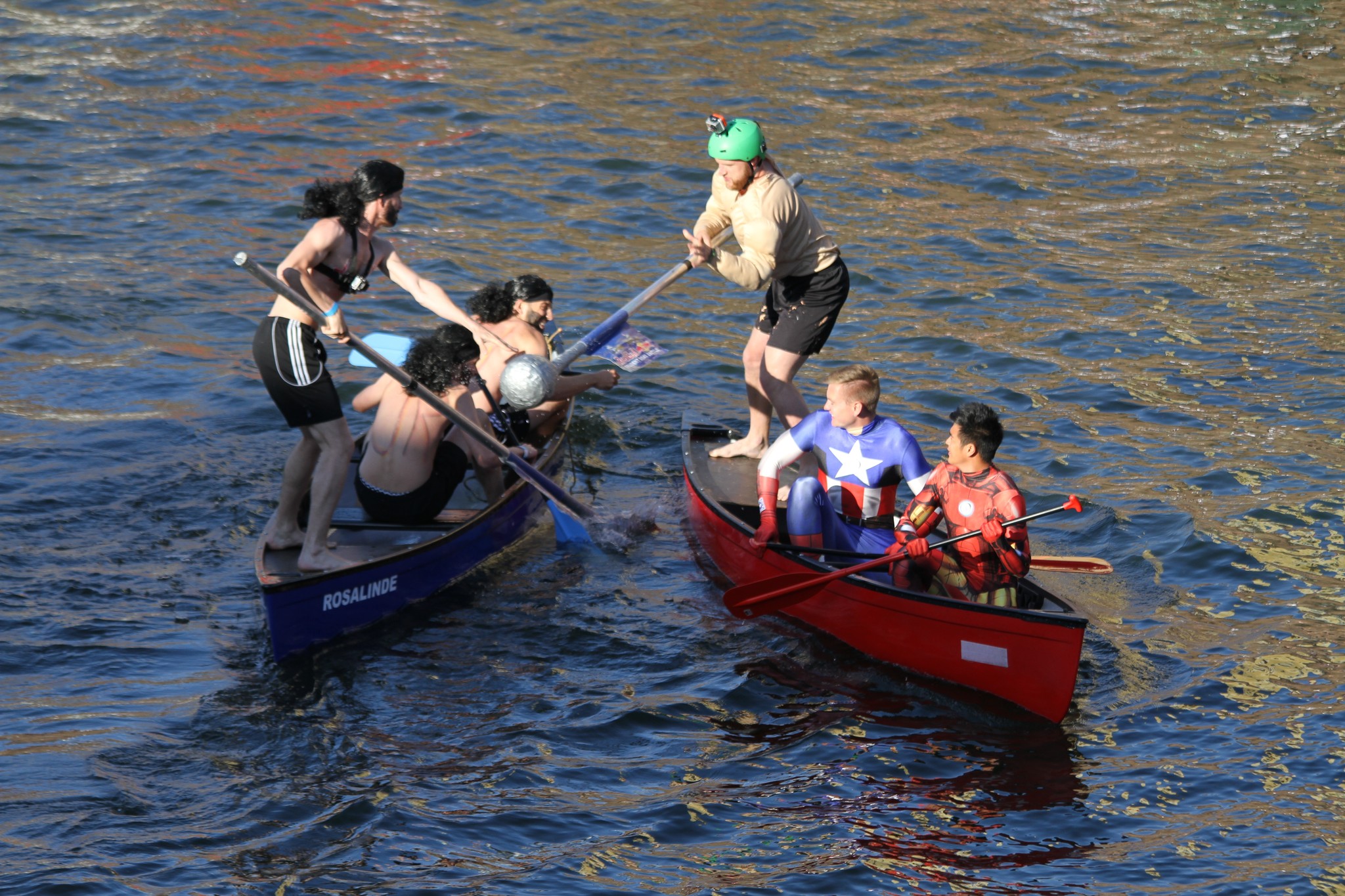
[[351, 275, 369, 291], [705, 114, 727, 134]]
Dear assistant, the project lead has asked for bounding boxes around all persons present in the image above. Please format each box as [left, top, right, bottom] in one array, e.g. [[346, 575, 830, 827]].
[[892, 402, 1030, 609], [751, 364, 944, 587], [682, 113, 850, 459], [252, 160, 519, 572], [351, 323, 538, 525], [459, 274, 620, 447]]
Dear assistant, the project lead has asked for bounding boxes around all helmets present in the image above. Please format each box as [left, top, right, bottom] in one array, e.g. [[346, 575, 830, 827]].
[[708, 118, 767, 162]]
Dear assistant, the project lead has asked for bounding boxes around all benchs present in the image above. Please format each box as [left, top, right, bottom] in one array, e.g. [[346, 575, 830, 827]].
[[297, 506, 483, 532]]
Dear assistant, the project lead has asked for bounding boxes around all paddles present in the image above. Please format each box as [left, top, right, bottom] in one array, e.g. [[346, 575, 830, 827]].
[[746, 539, 1114, 575], [473, 369, 594, 545], [349, 332, 587, 376], [720, 492, 1083, 622]]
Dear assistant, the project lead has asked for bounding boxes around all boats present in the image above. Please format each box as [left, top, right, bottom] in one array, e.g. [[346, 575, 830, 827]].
[[678, 407, 1091, 727], [252, 313, 582, 676]]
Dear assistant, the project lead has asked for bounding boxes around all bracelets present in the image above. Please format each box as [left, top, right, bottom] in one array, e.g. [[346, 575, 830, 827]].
[[324, 302, 339, 317], [517, 444, 528, 459], [706, 248, 716, 263]]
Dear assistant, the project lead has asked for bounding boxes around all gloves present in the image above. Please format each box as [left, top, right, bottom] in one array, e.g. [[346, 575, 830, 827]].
[[752, 516, 780, 556], [979, 519, 1008, 544], [906, 538, 930, 558]]
